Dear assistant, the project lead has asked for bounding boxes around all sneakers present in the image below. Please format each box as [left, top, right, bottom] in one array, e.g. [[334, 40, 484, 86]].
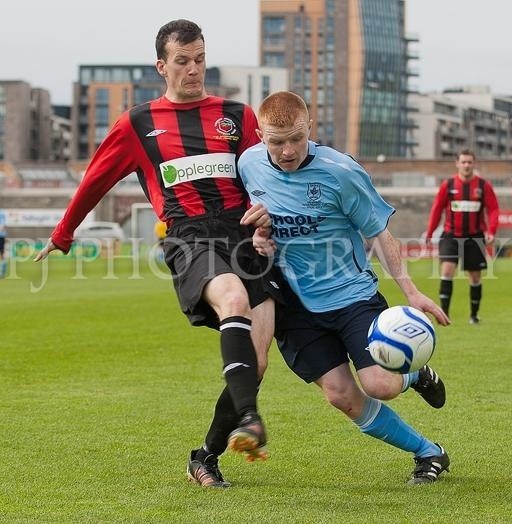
[[410, 364, 445, 408], [228, 421, 267, 461], [408, 443, 450, 486], [187, 450, 232, 489]]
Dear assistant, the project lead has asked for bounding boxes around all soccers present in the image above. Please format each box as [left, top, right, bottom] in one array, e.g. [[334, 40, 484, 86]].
[[368, 305, 436, 374]]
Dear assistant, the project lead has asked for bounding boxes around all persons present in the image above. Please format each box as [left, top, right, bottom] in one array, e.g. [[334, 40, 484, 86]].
[[235, 89, 453, 487], [31, 20, 288, 489], [0, 208, 9, 279], [423, 147, 499, 325]]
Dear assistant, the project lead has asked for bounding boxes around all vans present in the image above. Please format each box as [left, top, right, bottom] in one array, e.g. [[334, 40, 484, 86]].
[[70, 220, 128, 247]]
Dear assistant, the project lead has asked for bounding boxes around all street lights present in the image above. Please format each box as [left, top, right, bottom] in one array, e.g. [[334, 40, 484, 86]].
[[375, 153, 385, 187]]
